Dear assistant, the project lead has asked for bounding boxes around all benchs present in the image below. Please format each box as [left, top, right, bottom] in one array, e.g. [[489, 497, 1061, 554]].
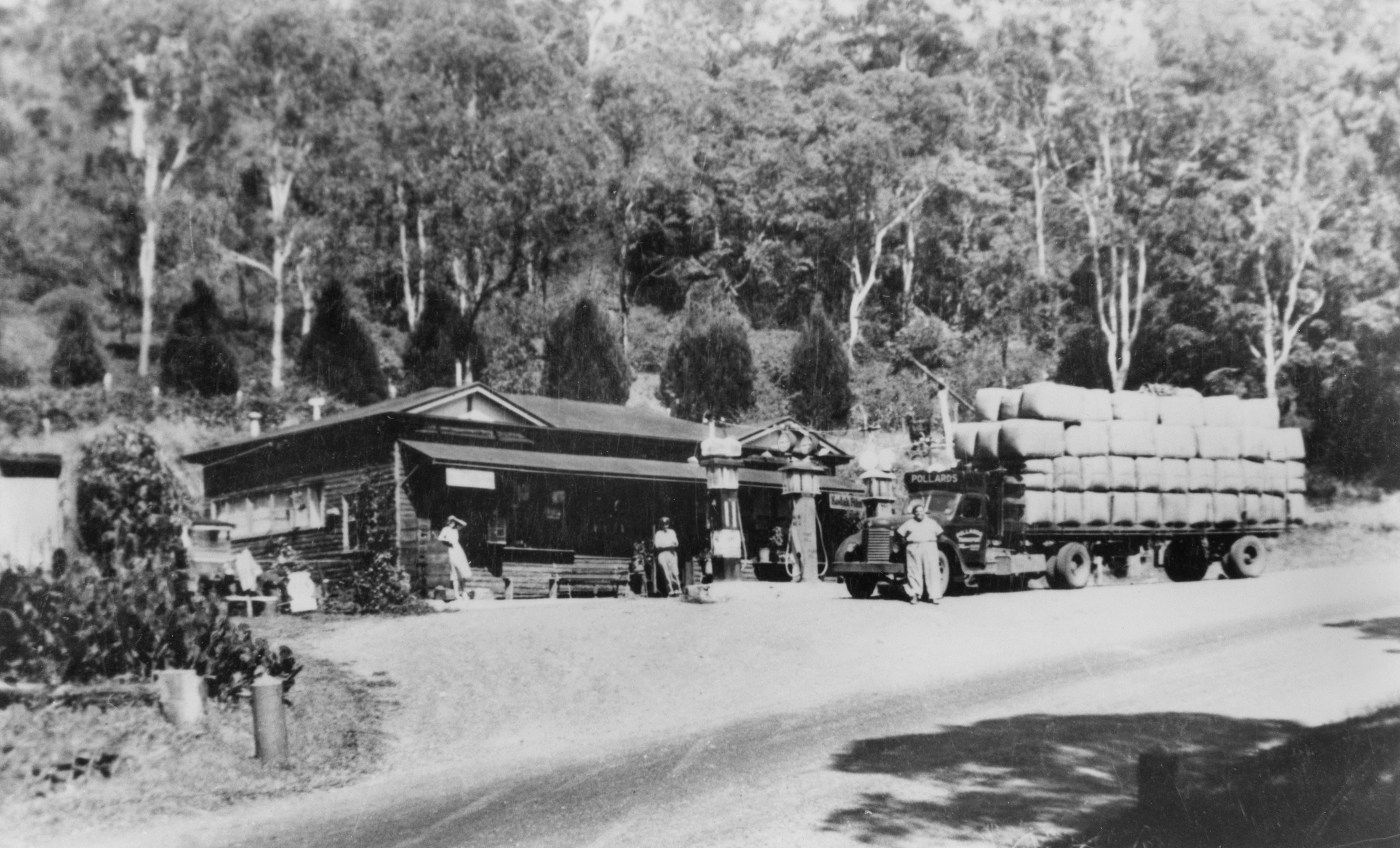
[[549, 564, 628, 601]]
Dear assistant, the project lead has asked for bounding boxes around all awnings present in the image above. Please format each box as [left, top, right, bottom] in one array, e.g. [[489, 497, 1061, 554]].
[[399, 439, 869, 494]]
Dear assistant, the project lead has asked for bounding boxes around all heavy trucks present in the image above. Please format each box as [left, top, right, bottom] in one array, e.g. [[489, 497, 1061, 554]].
[[827, 466, 1300, 600]]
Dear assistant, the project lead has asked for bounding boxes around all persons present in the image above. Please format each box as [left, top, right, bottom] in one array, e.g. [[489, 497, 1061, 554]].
[[438, 515, 473, 602], [897, 505, 946, 604], [653, 517, 683, 597]]
